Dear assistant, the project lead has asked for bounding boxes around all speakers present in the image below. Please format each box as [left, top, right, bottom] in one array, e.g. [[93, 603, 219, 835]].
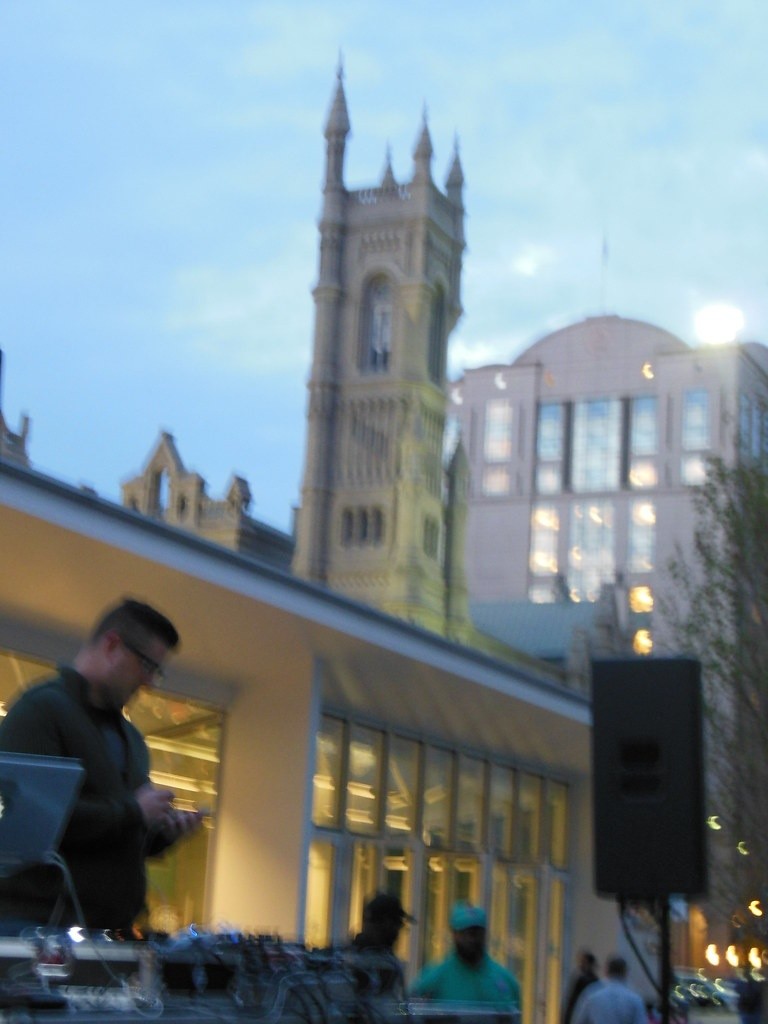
[[590, 658, 709, 906]]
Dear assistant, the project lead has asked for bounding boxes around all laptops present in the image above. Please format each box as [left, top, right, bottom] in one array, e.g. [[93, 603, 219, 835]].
[[0, 754, 84, 861]]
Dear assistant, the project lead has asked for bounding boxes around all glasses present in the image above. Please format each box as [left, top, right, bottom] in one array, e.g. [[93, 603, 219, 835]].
[[118, 634, 166, 680]]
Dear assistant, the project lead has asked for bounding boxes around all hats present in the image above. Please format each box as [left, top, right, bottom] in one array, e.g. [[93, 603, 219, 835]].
[[449, 900, 487, 929], [363, 895, 416, 926]]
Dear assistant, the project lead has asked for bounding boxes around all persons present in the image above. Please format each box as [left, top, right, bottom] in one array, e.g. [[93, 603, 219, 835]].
[[409, 902, 524, 1024], [351, 892, 408, 1024], [0, 597, 202, 1014], [560, 950, 661, 1024]]
